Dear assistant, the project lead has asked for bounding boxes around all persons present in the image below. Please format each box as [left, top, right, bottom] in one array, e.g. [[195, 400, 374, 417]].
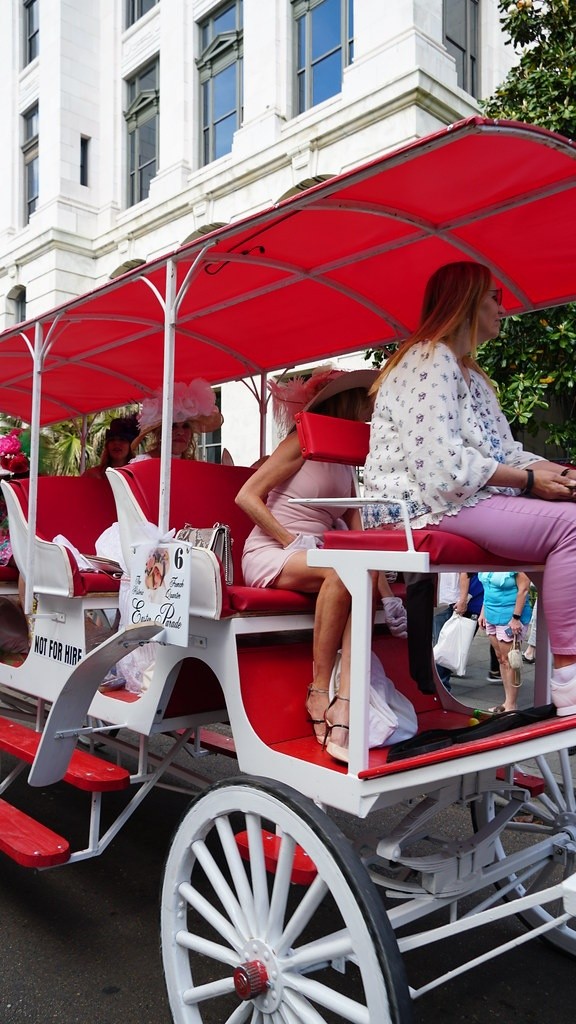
[[128, 379, 225, 464], [485, 645, 502, 682], [477, 572, 532, 714], [80, 417, 141, 480], [522, 599, 537, 664], [0, 428, 59, 647], [360, 261, 576, 716], [464, 573, 484, 636], [234, 365, 408, 762], [432, 572, 470, 692]]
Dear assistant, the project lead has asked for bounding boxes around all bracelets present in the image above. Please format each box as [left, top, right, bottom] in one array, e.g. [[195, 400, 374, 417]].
[[516, 469, 533, 497], [513, 614, 521, 620], [561, 469, 569, 476]]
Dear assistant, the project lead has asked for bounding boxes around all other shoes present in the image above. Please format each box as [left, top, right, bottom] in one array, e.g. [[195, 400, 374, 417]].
[[521, 653, 536, 665], [487, 705, 506, 713]]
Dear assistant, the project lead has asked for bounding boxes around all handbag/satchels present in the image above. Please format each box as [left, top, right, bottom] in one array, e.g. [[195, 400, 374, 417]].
[[507, 634, 524, 688], [433, 609, 478, 677], [81, 553, 123, 579], [175, 522, 234, 586], [329, 648, 417, 749]]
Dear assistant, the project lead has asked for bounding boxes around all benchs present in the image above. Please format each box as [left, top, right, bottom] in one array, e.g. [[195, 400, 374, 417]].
[[0, 411, 576, 794]]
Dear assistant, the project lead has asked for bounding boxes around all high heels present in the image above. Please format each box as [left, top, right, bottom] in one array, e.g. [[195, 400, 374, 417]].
[[320, 694, 349, 763], [306, 686, 329, 746]]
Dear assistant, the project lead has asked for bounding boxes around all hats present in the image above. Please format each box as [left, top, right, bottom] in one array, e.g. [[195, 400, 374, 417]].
[[105, 418, 139, 441], [268, 364, 381, 435], [131, 379, 224, 455]]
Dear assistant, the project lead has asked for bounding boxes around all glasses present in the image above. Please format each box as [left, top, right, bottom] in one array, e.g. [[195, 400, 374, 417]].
[[487, 287, 502, 305]]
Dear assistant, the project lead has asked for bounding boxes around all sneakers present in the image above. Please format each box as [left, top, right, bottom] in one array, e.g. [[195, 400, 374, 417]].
[[486, 671, 503, 683], [550, 676, 576, 716]]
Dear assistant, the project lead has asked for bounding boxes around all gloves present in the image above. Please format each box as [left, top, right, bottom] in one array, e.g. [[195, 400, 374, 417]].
[[282, 532, 323, 551], [381, 597, 407, 639]]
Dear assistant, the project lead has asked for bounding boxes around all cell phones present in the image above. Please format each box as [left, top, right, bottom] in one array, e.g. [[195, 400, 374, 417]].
[[504, 620, 525, 637]]
